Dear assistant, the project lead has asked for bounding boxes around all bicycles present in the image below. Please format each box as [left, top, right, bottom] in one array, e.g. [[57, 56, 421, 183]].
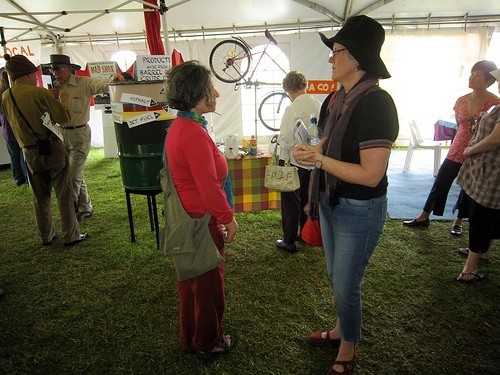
[[209, 29, 294, 131]]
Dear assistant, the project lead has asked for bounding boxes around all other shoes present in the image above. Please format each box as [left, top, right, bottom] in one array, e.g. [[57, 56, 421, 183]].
[[402, 218, 430, 227], [451, 223, 463, 235], [43, 231, 57, 246], [16, 179, 26, 186], [76, 211, 93, 221], [309, 330, 341, 343], [323, 347, 357, 375], [276, 240, 297, 253], [65, 232, 88, 246]]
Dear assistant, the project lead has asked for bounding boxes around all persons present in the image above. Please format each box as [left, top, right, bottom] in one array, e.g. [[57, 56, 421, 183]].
[[160, 60, 239, 360], [275, 71, 321, 252], [402, 58, 500, 234], [41, 54, 125, 220], [292, 15, 398, 375], [0, 70, 29, 186], [2, 54, 87, 247], [455, 68, 500, 283]]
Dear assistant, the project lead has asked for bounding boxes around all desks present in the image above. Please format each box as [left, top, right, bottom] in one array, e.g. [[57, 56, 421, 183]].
[[221, 144, 281, 211], [434, 115, 458, 144]]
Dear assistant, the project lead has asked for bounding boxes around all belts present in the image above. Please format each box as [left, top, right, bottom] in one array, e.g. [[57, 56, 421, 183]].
[[60, 124, 86, 129]]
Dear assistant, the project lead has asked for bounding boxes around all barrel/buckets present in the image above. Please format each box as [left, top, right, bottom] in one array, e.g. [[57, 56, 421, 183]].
[[106, 79, 173, 191]]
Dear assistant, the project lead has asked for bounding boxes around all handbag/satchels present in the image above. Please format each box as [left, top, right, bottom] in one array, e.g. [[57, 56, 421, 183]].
[[264, 165, 301, 192], [301, 215, 324, 247], [36, 139, 53, 156], [158, 168, 225, 283]]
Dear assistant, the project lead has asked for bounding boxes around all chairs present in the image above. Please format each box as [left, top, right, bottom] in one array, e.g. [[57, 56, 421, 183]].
[[404, 120, 443, 177]]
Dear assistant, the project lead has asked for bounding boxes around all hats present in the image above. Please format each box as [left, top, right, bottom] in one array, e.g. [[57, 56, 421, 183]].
[[317, 15, 391, 78], [489, 69, 500, 80], [6, 55, 39, 79], [47, 54, 81, 70]]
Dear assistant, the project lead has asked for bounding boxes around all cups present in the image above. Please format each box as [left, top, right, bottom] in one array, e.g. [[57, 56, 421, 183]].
[[243, 138, 251, 149]]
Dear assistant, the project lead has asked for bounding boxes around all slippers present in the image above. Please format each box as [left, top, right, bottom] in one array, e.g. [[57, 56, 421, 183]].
[[459, 248, 490, 260], [457, 271, 486, 283]]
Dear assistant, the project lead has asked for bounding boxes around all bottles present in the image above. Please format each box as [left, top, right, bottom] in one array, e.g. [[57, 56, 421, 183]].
[[219, 133, 239, 159], [250, 135, 257, 155], [307, 114, 323, 169]]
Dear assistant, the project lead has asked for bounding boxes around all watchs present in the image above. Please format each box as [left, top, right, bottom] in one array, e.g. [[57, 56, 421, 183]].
[[315, 155, 325, 169]]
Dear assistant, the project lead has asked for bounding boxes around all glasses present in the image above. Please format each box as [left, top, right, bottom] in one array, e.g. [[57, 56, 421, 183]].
[[329, 48, 347, 56], [48, 67, 65, 73]]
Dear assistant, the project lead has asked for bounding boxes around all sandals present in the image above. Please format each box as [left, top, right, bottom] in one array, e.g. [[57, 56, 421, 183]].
[[196, 335, 235, 358]]
